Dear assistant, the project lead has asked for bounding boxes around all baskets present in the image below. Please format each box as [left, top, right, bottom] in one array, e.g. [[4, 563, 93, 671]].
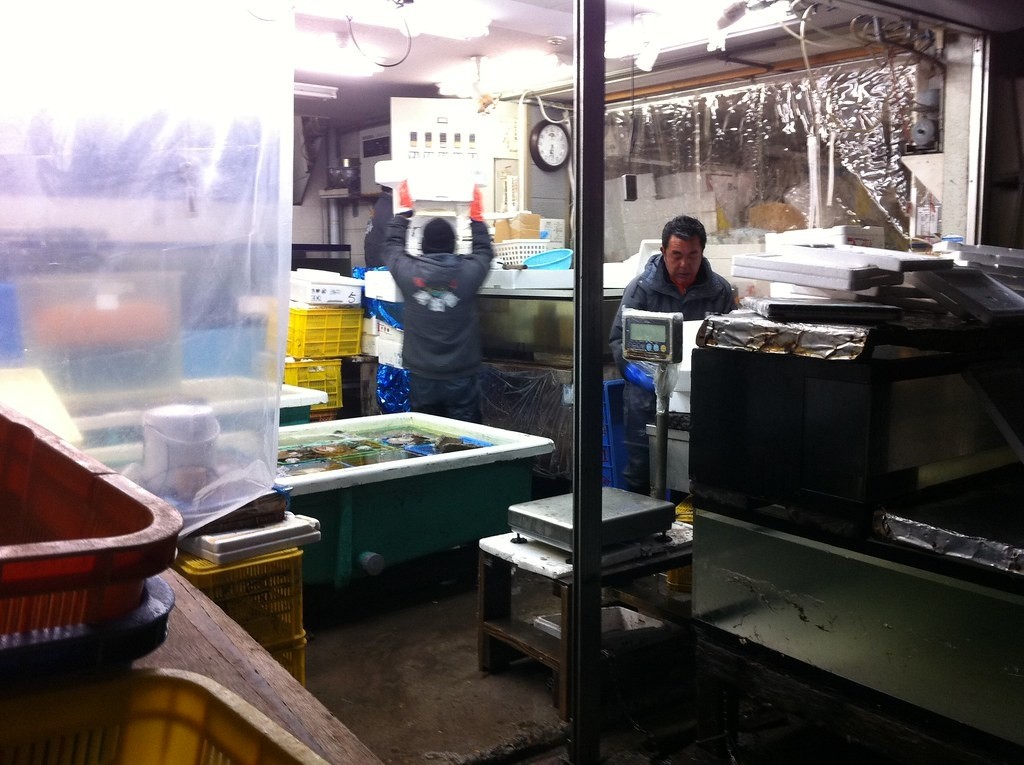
[[490, 239, 551, 267]]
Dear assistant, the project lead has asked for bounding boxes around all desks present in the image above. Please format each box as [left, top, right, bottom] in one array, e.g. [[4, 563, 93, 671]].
[[476, 522, 706, 720]]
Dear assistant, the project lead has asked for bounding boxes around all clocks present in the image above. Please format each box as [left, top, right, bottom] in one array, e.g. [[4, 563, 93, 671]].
[[530, 119, 568, 172]]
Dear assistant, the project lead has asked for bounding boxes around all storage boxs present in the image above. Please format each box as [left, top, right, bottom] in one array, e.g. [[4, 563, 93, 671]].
[[1, 264, 493, 764], [604, 171, 887, 301]]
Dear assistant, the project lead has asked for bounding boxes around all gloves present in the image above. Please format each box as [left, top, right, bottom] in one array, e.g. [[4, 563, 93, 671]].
[[393, 181, 417, 219], [624, 362, 655, 391], [468, 183, 484, 222]]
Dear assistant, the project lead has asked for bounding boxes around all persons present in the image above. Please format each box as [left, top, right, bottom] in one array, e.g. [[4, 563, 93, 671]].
[[364, 185, 394, 267], [609, 215, 738, 504], [383, 180, 495, 425]]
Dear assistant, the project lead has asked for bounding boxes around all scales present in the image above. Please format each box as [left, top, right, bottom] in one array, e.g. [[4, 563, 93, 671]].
[[507, 310, 683, 564]]
